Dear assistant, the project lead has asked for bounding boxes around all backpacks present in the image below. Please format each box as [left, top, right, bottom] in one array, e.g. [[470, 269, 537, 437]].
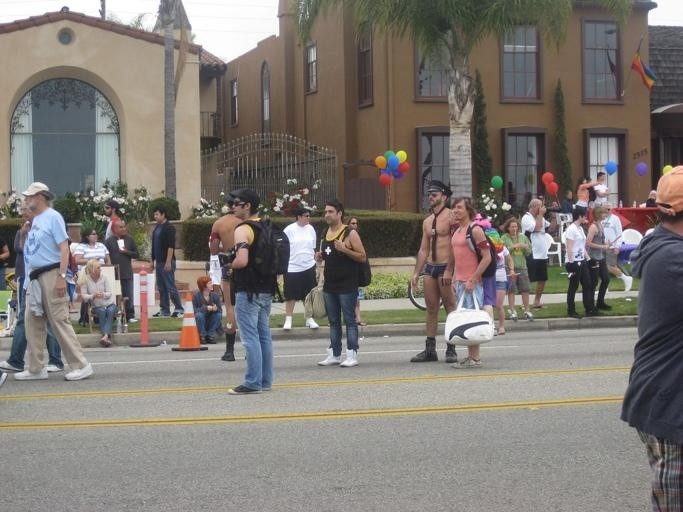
[[320, 224, 372, 288], [450, 222, 497, 277], [233, 217, 291, 275]]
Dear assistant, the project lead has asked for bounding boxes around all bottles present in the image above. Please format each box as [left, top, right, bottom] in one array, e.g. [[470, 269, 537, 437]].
[[117, 319, 123, 333]]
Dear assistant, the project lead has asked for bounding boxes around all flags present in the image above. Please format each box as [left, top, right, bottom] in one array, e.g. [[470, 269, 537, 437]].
[[628, 46, 658, 90]]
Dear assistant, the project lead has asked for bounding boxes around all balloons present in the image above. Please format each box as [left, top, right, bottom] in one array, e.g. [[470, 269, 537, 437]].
[[492, 176, 503, 189], [543, 173, 553, 185], [547, 181, 558, 194], [605, 160, 617, 176], [634, 160, 648, 178], [374, 148, 410, 188], [661, 164, 673, 176]]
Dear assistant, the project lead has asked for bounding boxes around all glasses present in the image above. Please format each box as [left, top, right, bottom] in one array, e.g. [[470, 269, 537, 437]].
[[233, 201, 247, 207]]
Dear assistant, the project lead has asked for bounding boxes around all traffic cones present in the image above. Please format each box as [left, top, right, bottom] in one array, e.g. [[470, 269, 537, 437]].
[[171, 290, 209, 351]]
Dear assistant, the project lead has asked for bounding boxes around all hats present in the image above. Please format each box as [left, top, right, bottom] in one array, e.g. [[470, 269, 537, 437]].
[[427, 179, 452, 198], [20, 181, 55, 202], [229, 186, 261, 204], [654, 165, 683, 216]]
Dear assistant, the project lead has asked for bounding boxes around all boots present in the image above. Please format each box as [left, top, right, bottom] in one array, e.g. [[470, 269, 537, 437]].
[[221, 331, 237, 361], [340, 348, 359, 368], [317, 346, 342, 368]]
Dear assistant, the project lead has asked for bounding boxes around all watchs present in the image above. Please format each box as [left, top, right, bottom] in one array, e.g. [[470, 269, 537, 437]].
[[59, 273, 66, 278]]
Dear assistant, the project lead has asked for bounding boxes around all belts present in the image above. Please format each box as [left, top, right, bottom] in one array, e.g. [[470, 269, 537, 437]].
[[29, 262, 60, 281]]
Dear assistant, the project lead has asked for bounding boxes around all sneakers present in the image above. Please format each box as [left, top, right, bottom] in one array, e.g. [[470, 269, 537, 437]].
[[0, 360, 25, 372], [200, 336, 216, 345], [65, 363, 93, 381], [492, 273, 633, 337], [445, 350, 459, 363], [410, 350, 438, 364], [305, 317, 319, 329], [128, 312, 184, 324], [45, 364, 64, 372], [283, 315, 293, 330], [15, 369, 49, 380], [228, 383, 271, 395], [450, 356, 483, 369], [99, 336, 113, 347]]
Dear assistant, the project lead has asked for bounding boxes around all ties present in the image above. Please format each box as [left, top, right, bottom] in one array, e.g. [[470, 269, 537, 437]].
[[430, 205, 447, 261]]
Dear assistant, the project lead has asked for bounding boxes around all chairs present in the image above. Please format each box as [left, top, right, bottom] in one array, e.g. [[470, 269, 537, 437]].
[[543, 232, 562, 267], [79, 264, 129, 334]]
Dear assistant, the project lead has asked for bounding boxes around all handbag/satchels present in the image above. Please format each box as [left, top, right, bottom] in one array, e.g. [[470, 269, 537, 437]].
[[444, 288, 495, 346], [304, 284, 328, 320]]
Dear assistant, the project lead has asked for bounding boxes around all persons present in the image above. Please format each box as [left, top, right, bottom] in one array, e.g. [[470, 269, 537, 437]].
[[2, 182, 112, 381], [104, 218, 139, 323], [343, 214, 364, 326], [442, 196, 515, 369], [521, 173, 632, 318], [104, 200, 124, 239], [500, 219, 533, 321], [618, 163, 683, 512], [150, 206, 184, 319], [410, 180, 458, 363], [77, 259, 116, 348], [193, 188, 275, 392], [280, 208, 320, 330], [315, 200, 367, 366]]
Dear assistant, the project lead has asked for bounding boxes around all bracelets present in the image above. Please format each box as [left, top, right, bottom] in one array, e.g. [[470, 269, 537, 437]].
[[552, 217, 556, 219]]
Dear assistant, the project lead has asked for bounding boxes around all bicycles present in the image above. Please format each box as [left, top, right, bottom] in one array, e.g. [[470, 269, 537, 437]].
[[409, 272, 445, 311]]
